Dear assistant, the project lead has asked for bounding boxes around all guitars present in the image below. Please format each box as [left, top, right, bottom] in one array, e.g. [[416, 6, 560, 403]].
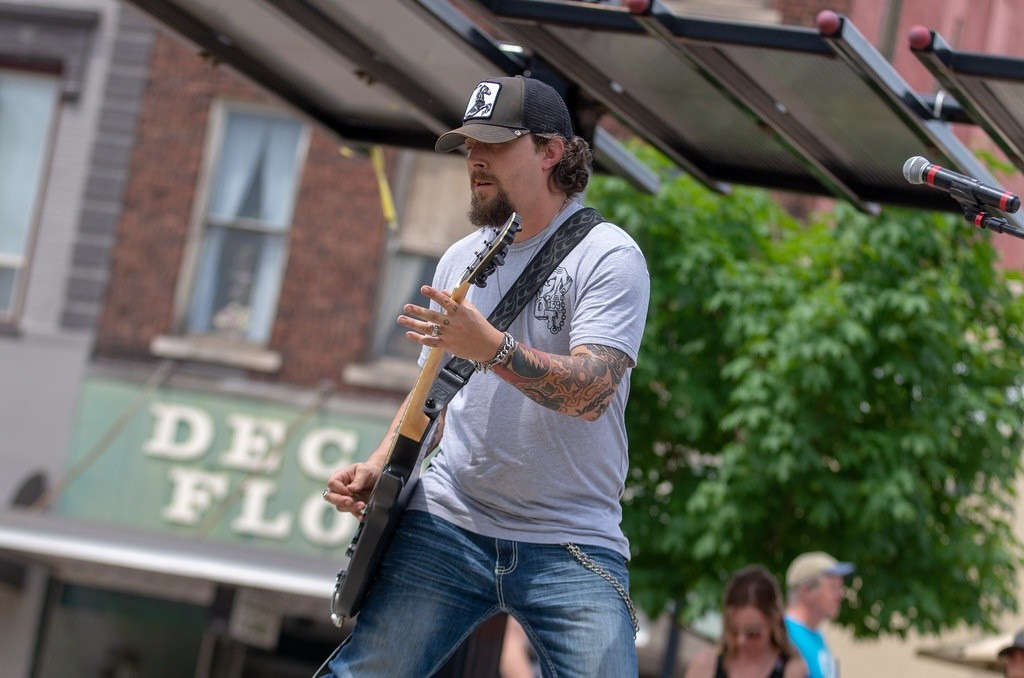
[[333, 212, 523, 617]]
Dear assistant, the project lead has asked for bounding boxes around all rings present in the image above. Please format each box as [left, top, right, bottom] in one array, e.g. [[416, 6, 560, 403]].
[[432, 325, 439, 337], [323, 490, 328, 495]]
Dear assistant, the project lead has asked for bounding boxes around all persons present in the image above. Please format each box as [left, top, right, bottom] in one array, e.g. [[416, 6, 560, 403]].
[[784, 548, 854, 678], [500, 614, 542, 678], [320, 76, 650, 678], [684, 563, 808, 678], [999, 627, 1024, 678]]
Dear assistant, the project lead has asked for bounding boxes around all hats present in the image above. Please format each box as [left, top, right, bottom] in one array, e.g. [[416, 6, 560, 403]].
[[787, 553, 855, 586], [436, 75, 572, 155], [1000, 625, 1023, 657]]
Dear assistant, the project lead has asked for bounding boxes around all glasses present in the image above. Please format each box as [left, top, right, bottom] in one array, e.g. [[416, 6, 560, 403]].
[[723, 623, 771, 642]]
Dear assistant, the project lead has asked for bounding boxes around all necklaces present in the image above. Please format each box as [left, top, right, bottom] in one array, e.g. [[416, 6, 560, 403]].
[[496, 198, 568, 300]]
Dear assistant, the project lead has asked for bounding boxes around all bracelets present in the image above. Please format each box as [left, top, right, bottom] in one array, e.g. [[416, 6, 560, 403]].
[[482, 332, 515, 374]]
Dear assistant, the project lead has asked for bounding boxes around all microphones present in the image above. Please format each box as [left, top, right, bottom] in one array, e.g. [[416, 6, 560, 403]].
[[903, 156, 1021, 214]]
